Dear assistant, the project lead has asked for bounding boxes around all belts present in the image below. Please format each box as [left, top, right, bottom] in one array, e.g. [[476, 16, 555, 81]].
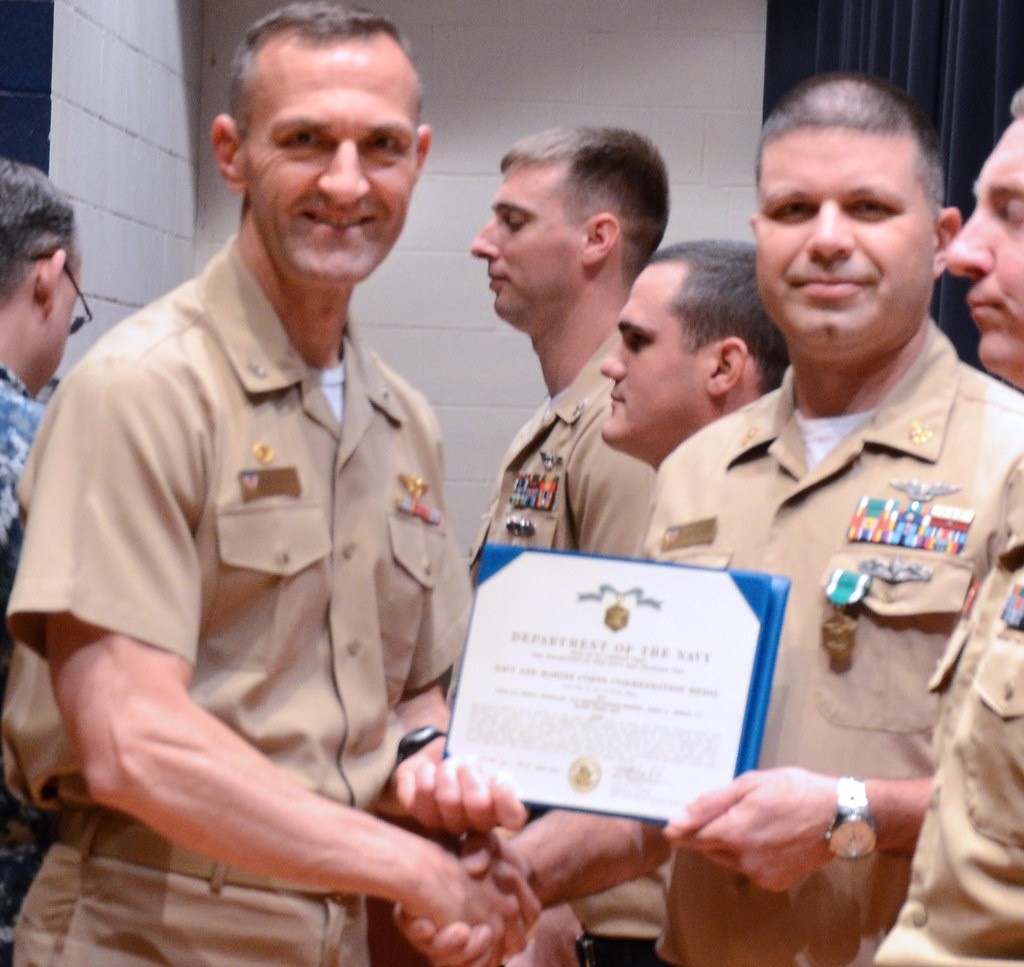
[[56, 801, 366, 896]]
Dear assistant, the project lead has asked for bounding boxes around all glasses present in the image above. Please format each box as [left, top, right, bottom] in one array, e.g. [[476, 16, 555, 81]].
[[57, 264, 94, 338]]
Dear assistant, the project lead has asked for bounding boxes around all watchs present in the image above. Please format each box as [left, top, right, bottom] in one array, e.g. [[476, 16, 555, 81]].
[[828, 778, 880, 857], [396, 721, 443, 759]]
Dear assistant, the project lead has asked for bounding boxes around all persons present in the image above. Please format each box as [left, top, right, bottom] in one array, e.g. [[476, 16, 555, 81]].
[[0, 3, 531, 967], [870, 83, 1024, 967], [469, 124, 656, 565], [0, 162, 94, 967], [395, 79, 1024, 967], [569, 240, 791, 966]]
